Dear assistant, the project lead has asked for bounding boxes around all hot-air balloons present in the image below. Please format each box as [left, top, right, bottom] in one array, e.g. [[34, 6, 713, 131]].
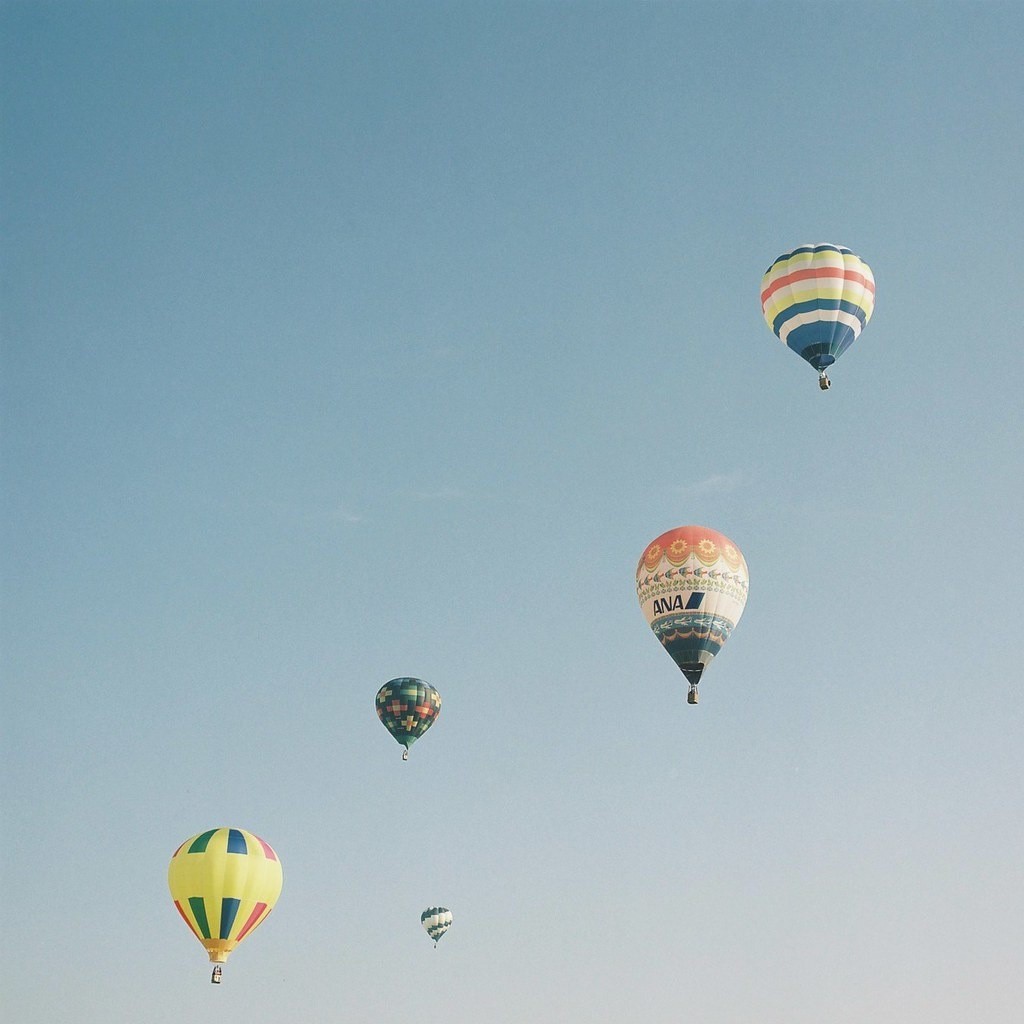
[[375, 677, 442, 761], [760, 243, 876, 391], [636, 525, 750, 705], [421, 906, 453, 949], [167, 828, 283, 983]]
